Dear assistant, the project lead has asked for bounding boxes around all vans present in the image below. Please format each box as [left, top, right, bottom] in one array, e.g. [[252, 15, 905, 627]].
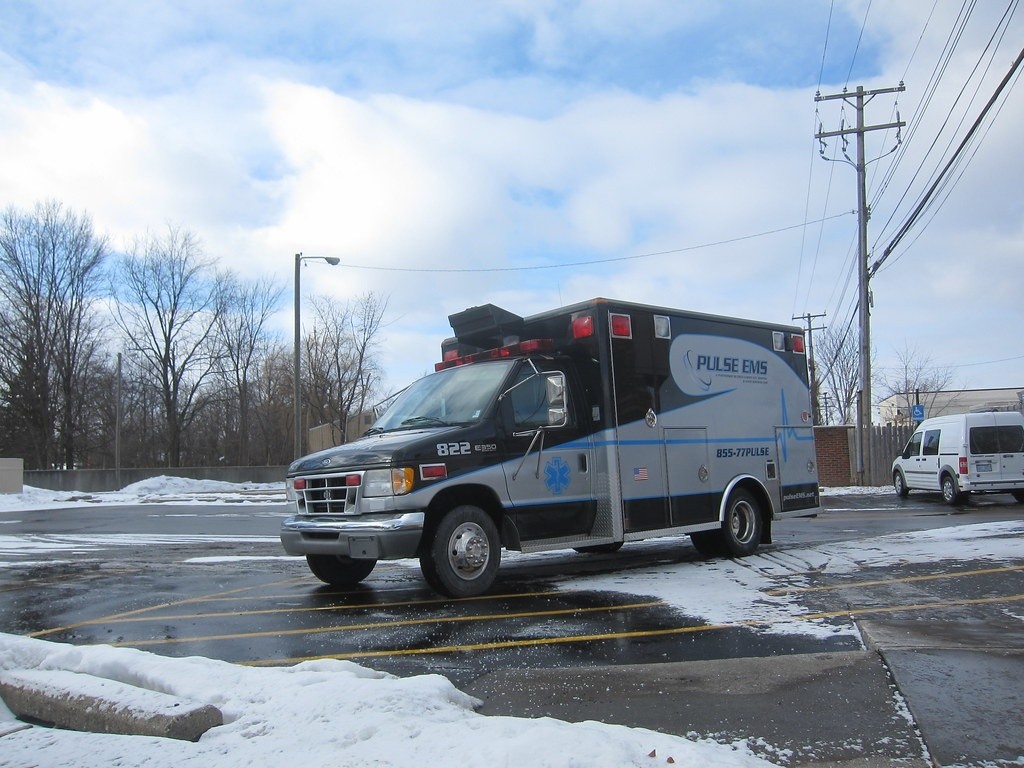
[[892, 412, 1024, 504]]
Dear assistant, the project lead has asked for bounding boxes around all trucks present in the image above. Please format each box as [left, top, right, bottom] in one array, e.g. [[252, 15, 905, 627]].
[[279, 296, 823, 597]]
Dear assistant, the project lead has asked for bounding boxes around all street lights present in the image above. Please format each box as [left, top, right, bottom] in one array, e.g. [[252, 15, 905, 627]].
[[294, 252, 341, 458]]
